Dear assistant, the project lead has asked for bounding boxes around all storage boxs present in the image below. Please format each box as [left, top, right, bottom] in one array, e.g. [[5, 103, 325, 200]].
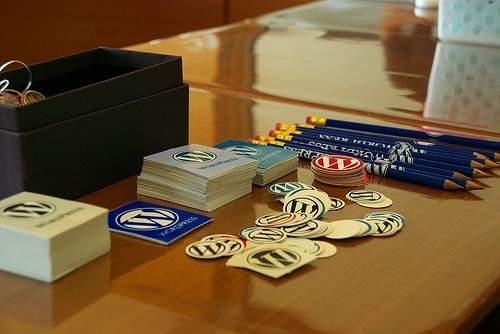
[[0, 46, 195, 193]]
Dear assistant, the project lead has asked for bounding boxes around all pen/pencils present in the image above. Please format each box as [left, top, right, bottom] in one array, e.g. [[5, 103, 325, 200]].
[[246, 116, 500, 193]]
